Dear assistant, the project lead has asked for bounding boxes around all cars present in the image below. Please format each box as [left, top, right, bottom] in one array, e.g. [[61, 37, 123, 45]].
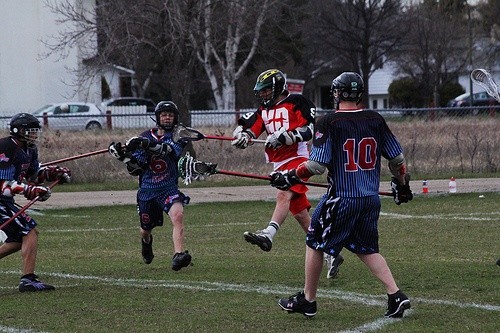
[[445, 91, 500, 116], [99, 95, 156, 115], [7, 101, 106, 132]]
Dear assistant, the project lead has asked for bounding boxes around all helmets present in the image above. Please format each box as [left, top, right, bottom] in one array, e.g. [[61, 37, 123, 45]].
[[10, 113, 41, 147], [253, 69, 287, 108], [331, 72, 364, 103], [155, 101, 179, 131]]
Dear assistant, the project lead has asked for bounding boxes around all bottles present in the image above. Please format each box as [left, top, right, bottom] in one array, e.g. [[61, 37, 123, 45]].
[[421, 178, 428, 193], [449, 176, 457, 193]]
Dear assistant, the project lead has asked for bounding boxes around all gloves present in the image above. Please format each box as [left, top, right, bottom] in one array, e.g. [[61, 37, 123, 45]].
[[38, 166, 71, 184], [108, 142, 129, 161], [269, 168, 302, 191], [266, 127, 294, 149], [231, 131, 254, 149], [391, 173, 413, 205], [11, 180, 51, 202]]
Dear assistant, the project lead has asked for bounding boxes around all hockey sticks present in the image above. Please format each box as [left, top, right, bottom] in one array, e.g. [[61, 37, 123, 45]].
[[176, 156, 395, 198], [38, 144, 126, 168], [171, 124, 267, 144], [0, 178, 62, 243]]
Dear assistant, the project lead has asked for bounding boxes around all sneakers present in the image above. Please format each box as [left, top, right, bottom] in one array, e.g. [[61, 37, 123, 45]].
[[244, 231, 272, 251], [141, 235, 154, 264], [19, 273, 55, 292], [172, 252, 191, 271], [327, 254, 344, 278], [384, 290, 411, 318], [278, 291, 317, 318]]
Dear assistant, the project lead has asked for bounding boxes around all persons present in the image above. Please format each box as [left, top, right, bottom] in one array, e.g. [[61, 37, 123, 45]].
[[108, 101, 216, 271], [0, 112, 71, 290], [232, 69, 344, 278], [269, 72, 412, 319]]
